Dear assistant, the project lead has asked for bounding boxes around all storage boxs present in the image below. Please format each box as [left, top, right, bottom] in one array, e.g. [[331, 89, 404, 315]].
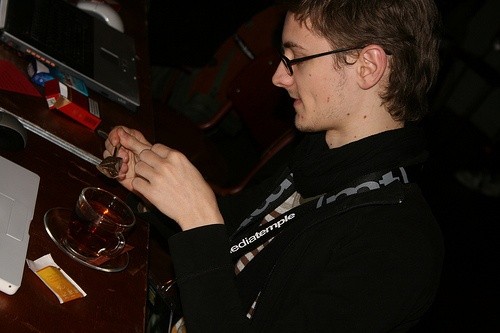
[[43, 78, 102, 131]]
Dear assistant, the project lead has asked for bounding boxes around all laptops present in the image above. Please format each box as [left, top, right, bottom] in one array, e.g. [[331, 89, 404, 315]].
[[0, 154, 41, 296], [0, 0, 141, 111]]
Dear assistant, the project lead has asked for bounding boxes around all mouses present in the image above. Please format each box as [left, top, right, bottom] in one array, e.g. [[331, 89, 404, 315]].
[[0, 111, 28, 150], [76, 0, 125, 34]]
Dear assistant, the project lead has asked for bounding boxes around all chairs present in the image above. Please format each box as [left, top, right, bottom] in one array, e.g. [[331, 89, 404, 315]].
[[156, 4, 297, 195]]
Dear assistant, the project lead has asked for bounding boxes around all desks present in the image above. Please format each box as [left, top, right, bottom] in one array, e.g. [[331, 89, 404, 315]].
[[0, 0, 150, 333]]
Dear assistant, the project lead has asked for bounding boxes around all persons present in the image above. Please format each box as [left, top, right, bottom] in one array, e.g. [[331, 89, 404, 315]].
[[104, 0, 446, 333]]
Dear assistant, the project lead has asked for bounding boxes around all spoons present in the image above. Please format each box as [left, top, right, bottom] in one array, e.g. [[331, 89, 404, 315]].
[[96, 123, 124, 179]]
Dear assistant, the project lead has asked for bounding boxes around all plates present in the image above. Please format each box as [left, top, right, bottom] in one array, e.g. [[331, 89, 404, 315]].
[[43, 207, 129, 272]]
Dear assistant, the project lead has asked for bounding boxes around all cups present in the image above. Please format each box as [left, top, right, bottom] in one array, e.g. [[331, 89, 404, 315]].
[[62, 188, 135, 262]]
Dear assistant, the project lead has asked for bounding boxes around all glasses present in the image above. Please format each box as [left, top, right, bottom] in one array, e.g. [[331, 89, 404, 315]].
[[280, 45, 393, 76]]
